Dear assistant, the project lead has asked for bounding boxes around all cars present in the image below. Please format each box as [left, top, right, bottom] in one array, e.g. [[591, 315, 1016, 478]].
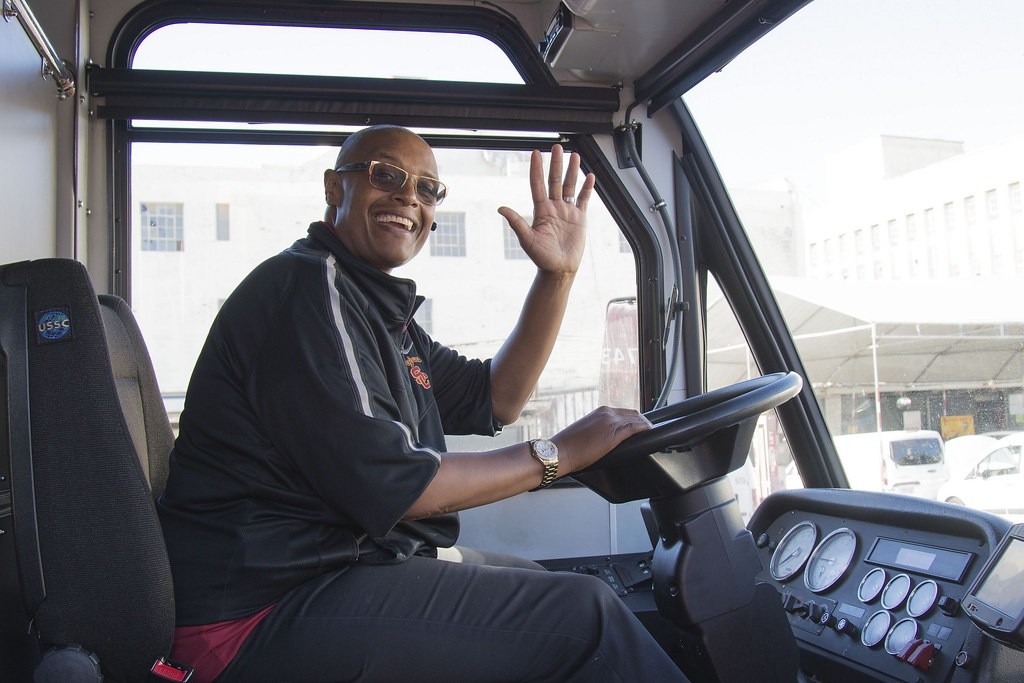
[[940, 430, 1024, 514]]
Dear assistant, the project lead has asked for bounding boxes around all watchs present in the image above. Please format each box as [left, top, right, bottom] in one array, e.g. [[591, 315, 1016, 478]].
[[527, 438, 559, 492]]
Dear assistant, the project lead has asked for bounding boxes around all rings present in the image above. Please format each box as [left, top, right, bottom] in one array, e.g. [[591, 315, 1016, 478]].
[[564, 196, 575, 203]]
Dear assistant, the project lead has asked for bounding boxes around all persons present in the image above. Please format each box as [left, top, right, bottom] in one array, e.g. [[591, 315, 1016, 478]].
[[159, 125, 701, 683]]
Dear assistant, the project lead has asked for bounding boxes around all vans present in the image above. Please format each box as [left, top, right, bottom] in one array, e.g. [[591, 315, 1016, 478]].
[[785, 428, 945, 501]]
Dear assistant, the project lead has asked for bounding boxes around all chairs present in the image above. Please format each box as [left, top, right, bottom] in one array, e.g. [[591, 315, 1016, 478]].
[[0, 257, 180, 683]]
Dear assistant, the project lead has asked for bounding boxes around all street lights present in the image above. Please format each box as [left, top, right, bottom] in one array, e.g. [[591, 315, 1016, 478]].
[[605, 296, 638, 556]]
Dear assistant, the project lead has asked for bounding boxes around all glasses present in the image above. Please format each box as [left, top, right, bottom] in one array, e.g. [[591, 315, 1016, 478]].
[[333, 160, 449, 206]]
